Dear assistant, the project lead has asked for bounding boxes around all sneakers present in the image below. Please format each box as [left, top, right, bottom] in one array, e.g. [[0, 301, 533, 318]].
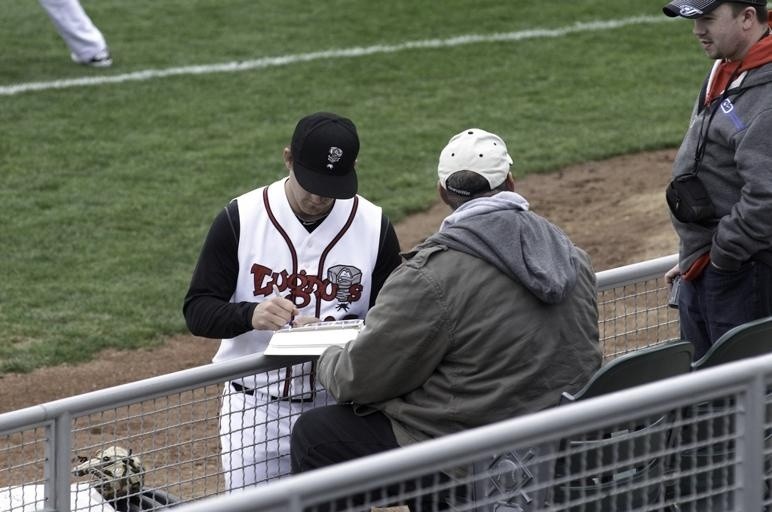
[[71, 48, 112, 66]]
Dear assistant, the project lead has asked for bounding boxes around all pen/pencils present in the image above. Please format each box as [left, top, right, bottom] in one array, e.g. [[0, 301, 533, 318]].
[[272, 284, 294, 327]]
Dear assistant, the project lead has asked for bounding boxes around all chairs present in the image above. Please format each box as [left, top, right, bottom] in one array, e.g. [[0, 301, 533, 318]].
[[448, 338, 693, 512], [114, 484, 185, 512], [687, 312, 771, 512]]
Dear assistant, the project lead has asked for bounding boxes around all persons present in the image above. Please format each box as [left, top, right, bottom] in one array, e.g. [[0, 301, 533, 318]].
[[663, 0, 772, 363], [37, 0, 114, 66], [290, 129, 603, 510], [181, 113, 402, 494]]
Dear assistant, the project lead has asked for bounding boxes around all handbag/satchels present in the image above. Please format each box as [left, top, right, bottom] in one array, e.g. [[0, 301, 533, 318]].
[[666, 172, 716, 224]]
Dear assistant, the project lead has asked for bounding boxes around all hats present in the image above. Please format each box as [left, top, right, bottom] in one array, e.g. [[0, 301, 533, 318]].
[[663, 0, 767, 18], [437, 128, 513, 196], [291, 113, 359, 199]]
